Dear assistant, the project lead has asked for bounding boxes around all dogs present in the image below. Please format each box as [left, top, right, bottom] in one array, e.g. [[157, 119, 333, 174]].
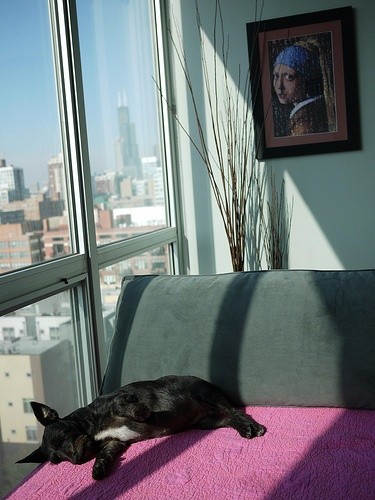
[[14, 374, 269, 480]]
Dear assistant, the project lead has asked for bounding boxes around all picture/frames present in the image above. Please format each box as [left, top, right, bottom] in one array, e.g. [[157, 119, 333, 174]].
[[244, 6, 361, 162]]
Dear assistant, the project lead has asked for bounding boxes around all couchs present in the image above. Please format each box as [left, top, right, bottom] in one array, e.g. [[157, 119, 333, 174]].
[[0, 267, 375, 499]]
